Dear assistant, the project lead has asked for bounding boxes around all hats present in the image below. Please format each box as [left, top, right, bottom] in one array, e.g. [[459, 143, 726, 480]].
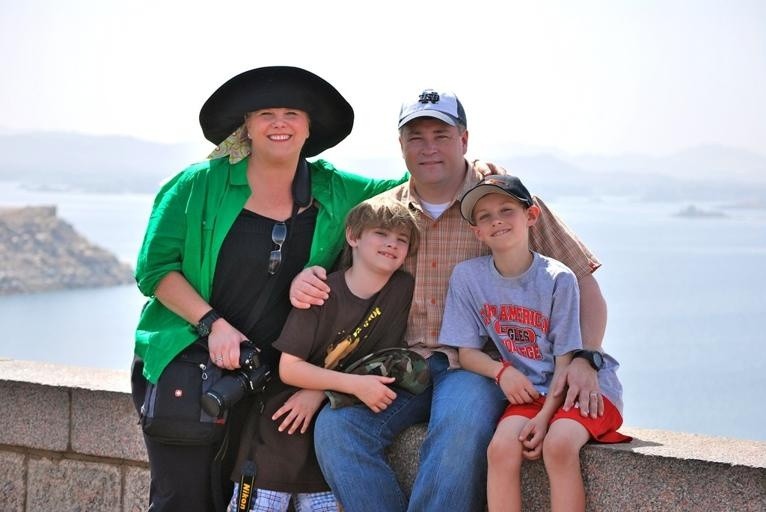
[[398, 90, 465, 130], [199, 66, 353, 157], [325, 348, 430, 409], [459, 175, 533, 225]]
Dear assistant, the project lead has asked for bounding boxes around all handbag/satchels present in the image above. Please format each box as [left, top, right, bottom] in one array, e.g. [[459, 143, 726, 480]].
[[141, 342, 229, 446]]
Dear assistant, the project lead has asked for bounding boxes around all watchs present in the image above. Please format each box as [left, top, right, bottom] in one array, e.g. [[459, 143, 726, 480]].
[[197, 309, 222, 337], [571, 349, 604, 369]]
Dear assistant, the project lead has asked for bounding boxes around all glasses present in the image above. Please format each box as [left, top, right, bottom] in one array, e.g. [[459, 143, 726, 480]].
[[267, 221, 287, 274]]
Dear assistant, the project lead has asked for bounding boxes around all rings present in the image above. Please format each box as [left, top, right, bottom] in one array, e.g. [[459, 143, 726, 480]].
[[216, 355, 223, 361], [591, 392, 596, 397]]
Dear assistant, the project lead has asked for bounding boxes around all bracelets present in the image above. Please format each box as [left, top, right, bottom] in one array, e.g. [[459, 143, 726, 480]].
[[494, 366, 510, 385]]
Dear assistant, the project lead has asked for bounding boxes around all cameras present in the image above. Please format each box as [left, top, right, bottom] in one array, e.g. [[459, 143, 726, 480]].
[[200, 340, 273, 417]]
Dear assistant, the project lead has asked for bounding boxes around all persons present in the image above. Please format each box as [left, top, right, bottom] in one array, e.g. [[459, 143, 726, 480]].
[[134, 64, 507, 512], [289, 87, 607, 512], [224, 196, 421, 512], [438, 173, 633, 512]]
